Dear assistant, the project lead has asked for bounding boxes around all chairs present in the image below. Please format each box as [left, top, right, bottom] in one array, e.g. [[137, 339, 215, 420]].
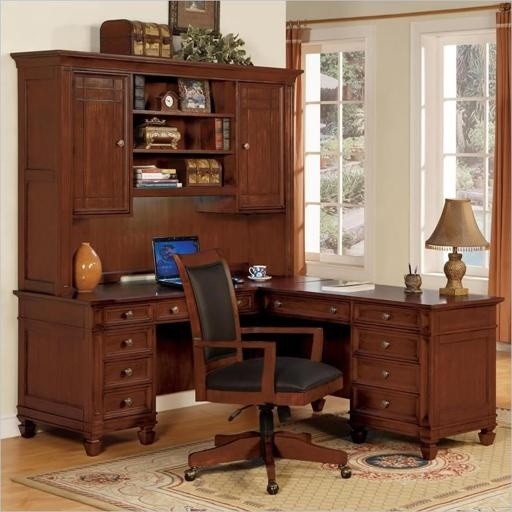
[[173, 249, 350, 494]]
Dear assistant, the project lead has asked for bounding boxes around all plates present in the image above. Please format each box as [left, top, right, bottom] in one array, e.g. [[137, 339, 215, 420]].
[[248, 276, 272, 282]]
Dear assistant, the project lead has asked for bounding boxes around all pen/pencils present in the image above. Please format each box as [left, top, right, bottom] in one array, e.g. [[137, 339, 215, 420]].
[[409, 264, 411, 274], [414, 264, 418, 273]]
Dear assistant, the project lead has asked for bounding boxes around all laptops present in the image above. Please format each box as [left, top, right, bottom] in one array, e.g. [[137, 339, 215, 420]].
[[151, 234, 238, 289]]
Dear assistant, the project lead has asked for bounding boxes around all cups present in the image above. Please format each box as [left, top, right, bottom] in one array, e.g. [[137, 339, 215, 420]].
[[249, 266, 267, 277]]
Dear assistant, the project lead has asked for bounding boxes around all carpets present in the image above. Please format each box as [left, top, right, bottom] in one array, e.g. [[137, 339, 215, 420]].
[[10, 406, 512, 512]]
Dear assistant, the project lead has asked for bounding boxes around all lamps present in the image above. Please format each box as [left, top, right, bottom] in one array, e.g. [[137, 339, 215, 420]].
[[425, 199, 489, 297]]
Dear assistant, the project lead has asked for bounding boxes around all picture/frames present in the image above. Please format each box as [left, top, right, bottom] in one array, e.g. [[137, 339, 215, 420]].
[[178, 78, 211, 113], [169, 0, 220, 37]]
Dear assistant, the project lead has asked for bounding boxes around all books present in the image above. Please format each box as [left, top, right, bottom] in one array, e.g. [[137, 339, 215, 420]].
[[322, 282, 375, 293], [132, 165, 183, 188], [201, 119, 230, 149]]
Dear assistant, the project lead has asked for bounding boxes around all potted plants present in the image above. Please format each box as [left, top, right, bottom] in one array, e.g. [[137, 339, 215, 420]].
[[405, 264, 423, 292]]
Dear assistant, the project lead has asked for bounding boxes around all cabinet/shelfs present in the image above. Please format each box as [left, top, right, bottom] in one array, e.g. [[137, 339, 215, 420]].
[[10, 51, 304, 299], [13, 277, 504, 459]]
